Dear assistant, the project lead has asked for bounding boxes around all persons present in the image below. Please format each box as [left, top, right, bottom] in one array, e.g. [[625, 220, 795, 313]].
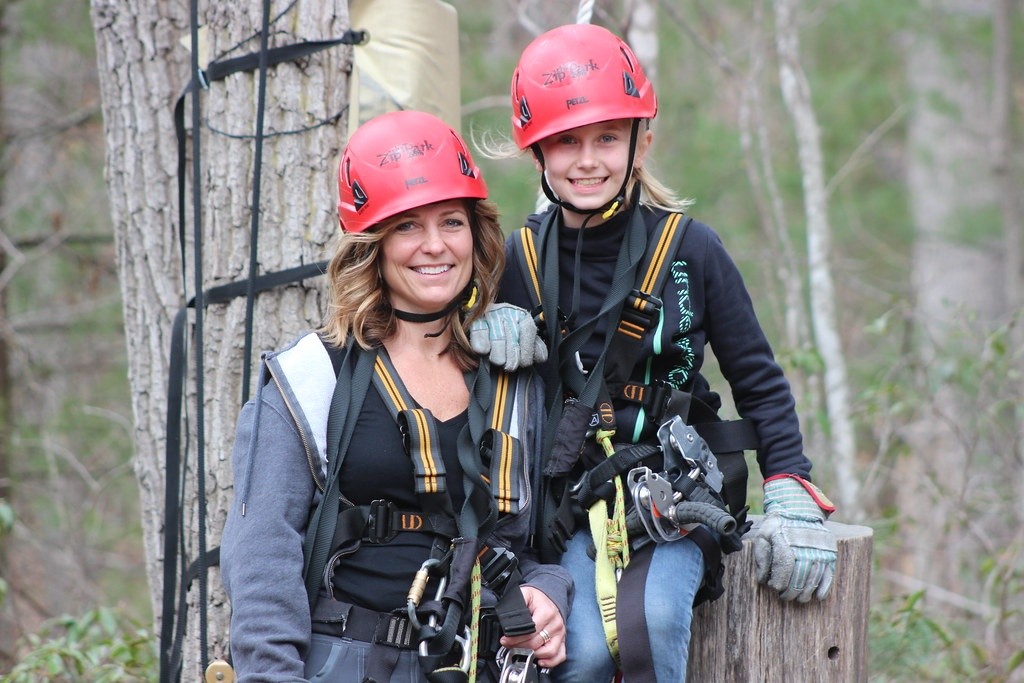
[[464, 27, 838, 683], [220, 109, 576, 682]]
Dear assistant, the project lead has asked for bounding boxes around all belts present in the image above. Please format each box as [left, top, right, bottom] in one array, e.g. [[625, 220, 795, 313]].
[[312, 597, 419, 651]]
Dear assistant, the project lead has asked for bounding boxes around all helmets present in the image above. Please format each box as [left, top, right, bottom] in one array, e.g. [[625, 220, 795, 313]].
[[338, 110, 488, 234], [511, 24, 658, 150]]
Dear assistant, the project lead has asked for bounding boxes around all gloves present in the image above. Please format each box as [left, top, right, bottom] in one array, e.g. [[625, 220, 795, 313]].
[[755, 474, 838, 603], [469, 302, 548, 372]]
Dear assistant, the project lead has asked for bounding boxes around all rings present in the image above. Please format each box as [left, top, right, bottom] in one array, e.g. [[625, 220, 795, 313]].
[[539, 629, 551, 644]]
